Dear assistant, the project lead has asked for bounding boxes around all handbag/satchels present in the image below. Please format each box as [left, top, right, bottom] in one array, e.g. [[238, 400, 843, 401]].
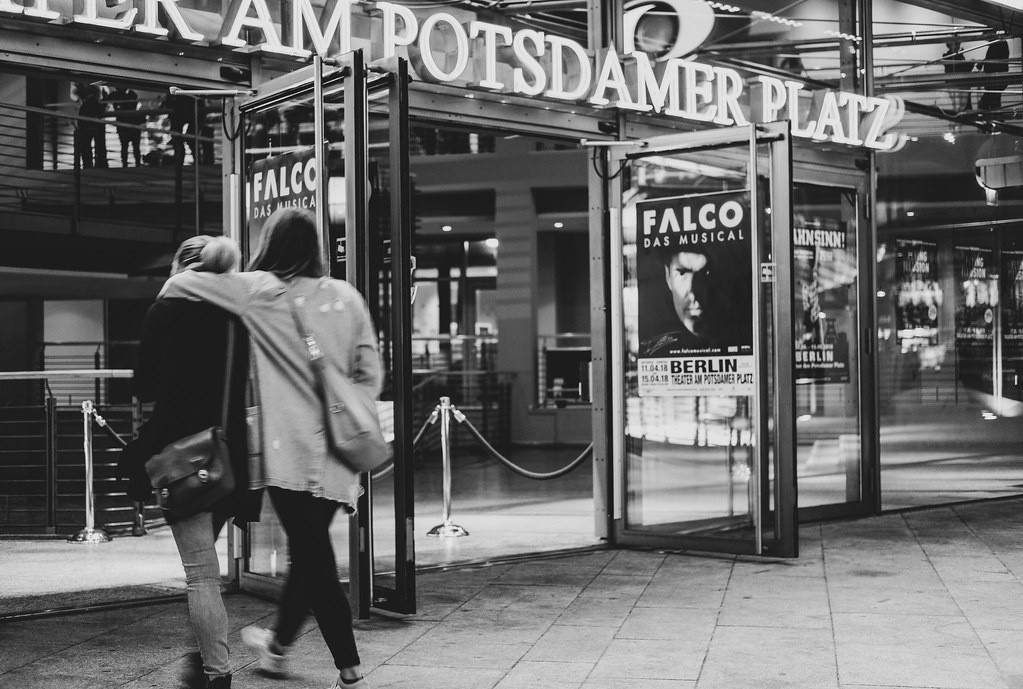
[[144, 425, 236, 525], [314, 356, 392, 472]]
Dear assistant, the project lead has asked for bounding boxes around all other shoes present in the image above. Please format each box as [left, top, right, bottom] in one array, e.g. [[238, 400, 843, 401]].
[[205, 672, 232, 689], [242, 625, 290, 674], [327, 672, 371, 689]]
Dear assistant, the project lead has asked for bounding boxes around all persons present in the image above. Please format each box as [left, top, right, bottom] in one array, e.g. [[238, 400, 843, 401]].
[[59, 76, 218, 171], [134, 236, 243, 689], [941, 28, 1013, 122], [154, 206, 384, 689], [777, 40, 806, 75], [636, 237, 754, 356]]
